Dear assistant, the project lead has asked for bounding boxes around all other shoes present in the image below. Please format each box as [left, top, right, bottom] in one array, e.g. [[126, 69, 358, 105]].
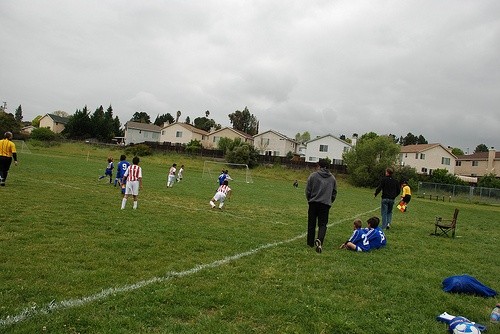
[[0, 181, 5, 186], [219, 207, 222, 209], [314, 239, 322, 252], [211, 205, 216, 209]]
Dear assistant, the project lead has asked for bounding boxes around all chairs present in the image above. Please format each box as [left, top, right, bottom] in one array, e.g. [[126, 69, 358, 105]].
[[435, 208, 459, 239]]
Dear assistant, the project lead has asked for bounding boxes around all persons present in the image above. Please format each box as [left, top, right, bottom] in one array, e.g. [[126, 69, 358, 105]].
[[218, 170, 234, 186], [0, 132, 17, 185], [340, 219, 370, 252], [372, 217, 386, 245], [373, 168, 400, 230], [210, 180, 232, 210], [167, 164, 177, 188], [117, 155, 131, 195], [361, 218, 381, 249], [305, 158, 338, 253], [99, 158, 114, 183], [400, 184, 411, 213], [293, 179, 298, 188], [120, 157, 142, 210], [176, 165, 185, 184]]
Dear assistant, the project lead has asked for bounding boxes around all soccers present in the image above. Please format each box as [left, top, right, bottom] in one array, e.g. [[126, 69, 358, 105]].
[[454, 322, 480, 334]]
